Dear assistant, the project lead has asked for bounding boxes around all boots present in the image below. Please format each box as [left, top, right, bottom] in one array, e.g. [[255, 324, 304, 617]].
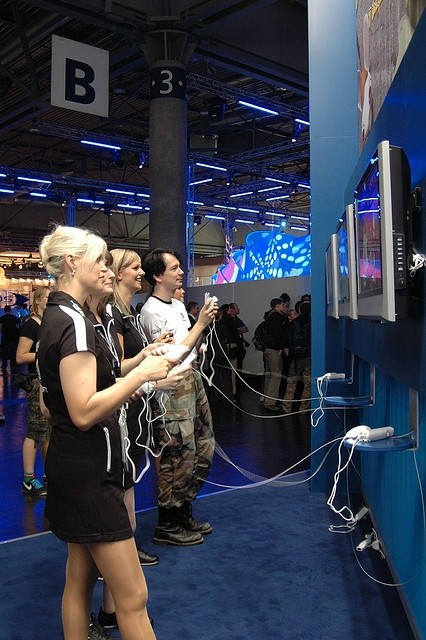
[[178, 501, 212, 533], [152, 505, 204, 546]]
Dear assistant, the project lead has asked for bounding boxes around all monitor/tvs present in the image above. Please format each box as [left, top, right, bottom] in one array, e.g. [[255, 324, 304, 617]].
[[354, 140, 416, 322], [325, 234, 337, 320], [336, 204, 356, 323]]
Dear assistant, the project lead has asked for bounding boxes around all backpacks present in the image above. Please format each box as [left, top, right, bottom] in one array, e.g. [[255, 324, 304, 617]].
[[251, 310, 273, 352]]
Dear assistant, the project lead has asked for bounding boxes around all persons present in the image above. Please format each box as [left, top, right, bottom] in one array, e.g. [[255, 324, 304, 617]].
[[97, 247, 159, 579], [184, 299, 220, 409], [221, 291, 313, 416], [150, 287, 186, 478], [136, 249, 220, 547], [37, 225, 178, 640], [0, 285, 52, 498], [90, 249, 144, 639]]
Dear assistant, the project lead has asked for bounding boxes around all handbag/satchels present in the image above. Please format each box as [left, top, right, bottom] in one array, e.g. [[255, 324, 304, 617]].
[[14, 372, 34, 393]]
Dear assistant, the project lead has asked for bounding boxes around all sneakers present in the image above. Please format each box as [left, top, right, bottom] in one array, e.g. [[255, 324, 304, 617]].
[[21, 478, 47, 495], [136, 549, 159, 566], [86, 612, 114, 640], [265, 405, 282, 412], [98, 606, 154, 629]]
